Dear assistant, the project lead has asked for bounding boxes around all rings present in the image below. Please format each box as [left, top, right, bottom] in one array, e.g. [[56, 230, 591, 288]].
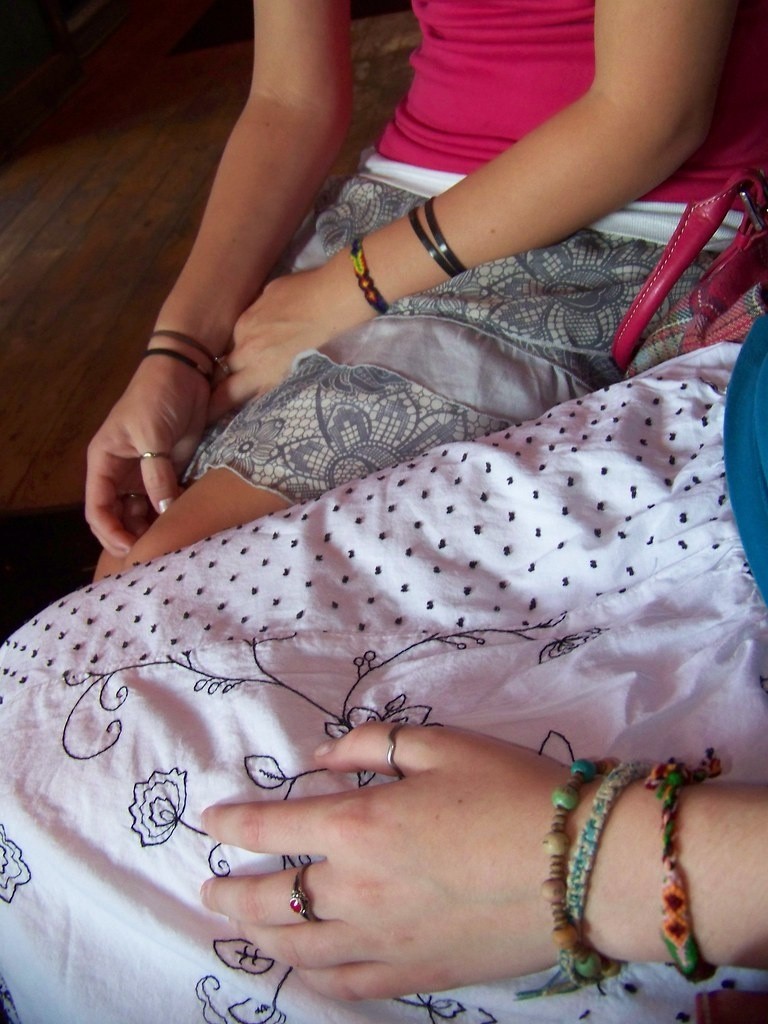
[[215, 355, 233, 376], [140, 453, 176, 463], [287, 858, 323, 926], [384, 721, 418, 778], [122, 490, 150, 503]]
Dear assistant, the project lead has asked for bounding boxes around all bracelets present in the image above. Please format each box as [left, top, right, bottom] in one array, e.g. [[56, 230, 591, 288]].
[[349, 236, 390, 314], [407, 206, 459, 278], [426, 195, 468, 277], [514, 761, 657, 1002], [149, 327, 217, 365], [642, 743, 725, 982], [538, 754, 622, 980], [142, 347, 215, 397]]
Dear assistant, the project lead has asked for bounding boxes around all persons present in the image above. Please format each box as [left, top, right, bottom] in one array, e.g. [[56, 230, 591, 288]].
[[81, 0, 768, 585], [0, 296, 768, 1024]]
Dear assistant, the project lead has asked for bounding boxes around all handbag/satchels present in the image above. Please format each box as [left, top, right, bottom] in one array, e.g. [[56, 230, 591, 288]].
[[612, 167, 768, 381]]
